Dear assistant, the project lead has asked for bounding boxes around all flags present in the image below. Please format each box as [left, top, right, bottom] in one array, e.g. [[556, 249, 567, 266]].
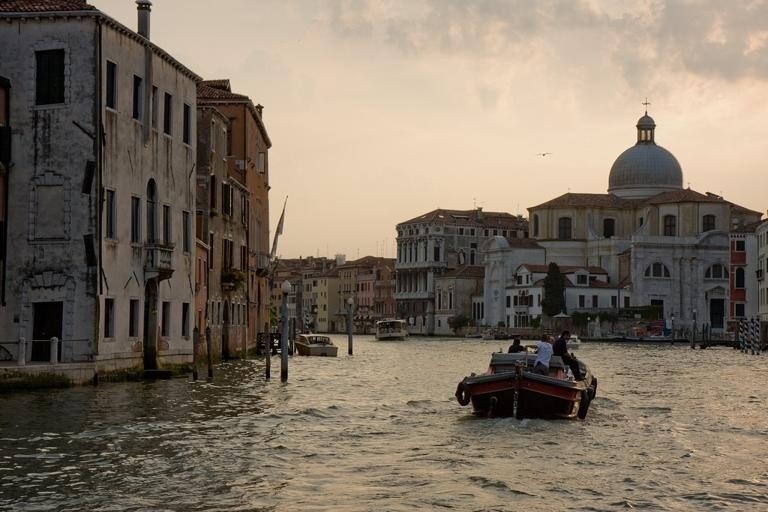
[[271, 208, 285, 256]]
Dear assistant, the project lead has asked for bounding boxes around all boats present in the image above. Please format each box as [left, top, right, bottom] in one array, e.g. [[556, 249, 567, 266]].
[[376, 318, 410, 341], [294, 332, 338, 357], [455, 344, 597, 421]]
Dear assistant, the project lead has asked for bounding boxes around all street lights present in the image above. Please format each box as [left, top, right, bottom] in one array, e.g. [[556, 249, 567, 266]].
[[346, 297, 354, 354], [280, 279, 292, 379]]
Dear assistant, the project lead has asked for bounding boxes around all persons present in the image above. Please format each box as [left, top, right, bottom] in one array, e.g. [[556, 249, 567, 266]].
[[554, 329, 587, 380], [508, 338, 524, 352], [526, 335, 554, 376]]
